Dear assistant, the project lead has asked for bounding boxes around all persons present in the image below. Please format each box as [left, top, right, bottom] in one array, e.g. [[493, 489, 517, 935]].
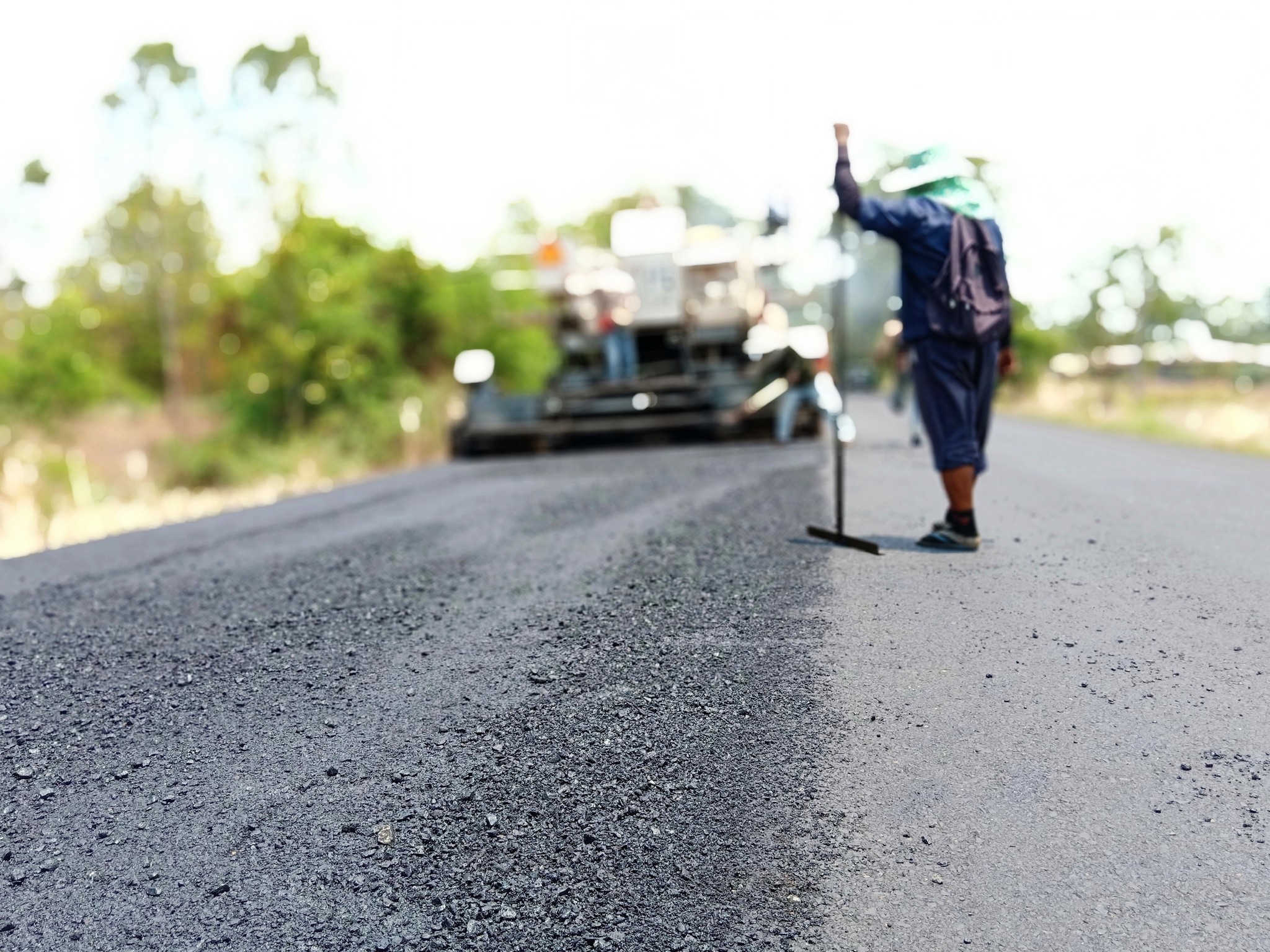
[[832, 121, 1016, 550]]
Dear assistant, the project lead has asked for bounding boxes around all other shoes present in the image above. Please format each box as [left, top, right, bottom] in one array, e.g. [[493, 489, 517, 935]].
[[916, 530, 979, 550], [934, 521, 954, 533]]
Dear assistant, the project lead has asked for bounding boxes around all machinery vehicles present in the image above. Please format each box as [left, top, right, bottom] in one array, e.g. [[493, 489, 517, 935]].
[[450, 249, 828, 461]]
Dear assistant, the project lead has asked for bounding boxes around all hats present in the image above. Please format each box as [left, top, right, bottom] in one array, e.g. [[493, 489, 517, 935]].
[[879, 146, 976, 193]]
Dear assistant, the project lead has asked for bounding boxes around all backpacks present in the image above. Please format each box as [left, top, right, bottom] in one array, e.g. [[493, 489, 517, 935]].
[[927, 212, 1006, 340]]
